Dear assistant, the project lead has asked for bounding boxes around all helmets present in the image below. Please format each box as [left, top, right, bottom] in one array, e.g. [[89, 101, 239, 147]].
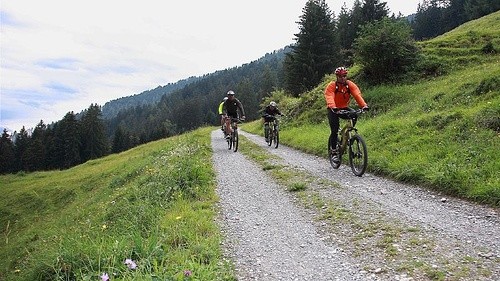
[[270, 101, 277, 107], [335, 67, 348, 75], [227, 91, 235, 95], [223, 97, 228, 100]]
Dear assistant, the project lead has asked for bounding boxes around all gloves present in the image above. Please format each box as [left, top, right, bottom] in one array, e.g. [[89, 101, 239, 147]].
[[364, 106, 369, 111], [332, 108, 339, 113]]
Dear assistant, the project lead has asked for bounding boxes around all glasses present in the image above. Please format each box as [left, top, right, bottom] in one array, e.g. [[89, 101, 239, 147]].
[[339, 75, 347, 78], [228, 94, 233, 96]]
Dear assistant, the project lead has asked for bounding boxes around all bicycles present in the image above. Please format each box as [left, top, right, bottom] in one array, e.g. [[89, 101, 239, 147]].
[[267, 114, 284, 149], [220, 113, 245, 152], [327, 108, 368, 176]]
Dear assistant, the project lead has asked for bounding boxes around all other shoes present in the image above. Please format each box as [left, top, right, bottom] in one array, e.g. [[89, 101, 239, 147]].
[[265, 138, 269, 142], [331, 152, 341, 163], [226, 135, 230, 139]]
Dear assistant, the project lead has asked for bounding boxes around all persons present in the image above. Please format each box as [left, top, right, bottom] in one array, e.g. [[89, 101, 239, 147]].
[[325, 67, 369, 163], [222, 91, 246, 139], [261, 101, 285, 142], [218, 97, 229, 131]]
